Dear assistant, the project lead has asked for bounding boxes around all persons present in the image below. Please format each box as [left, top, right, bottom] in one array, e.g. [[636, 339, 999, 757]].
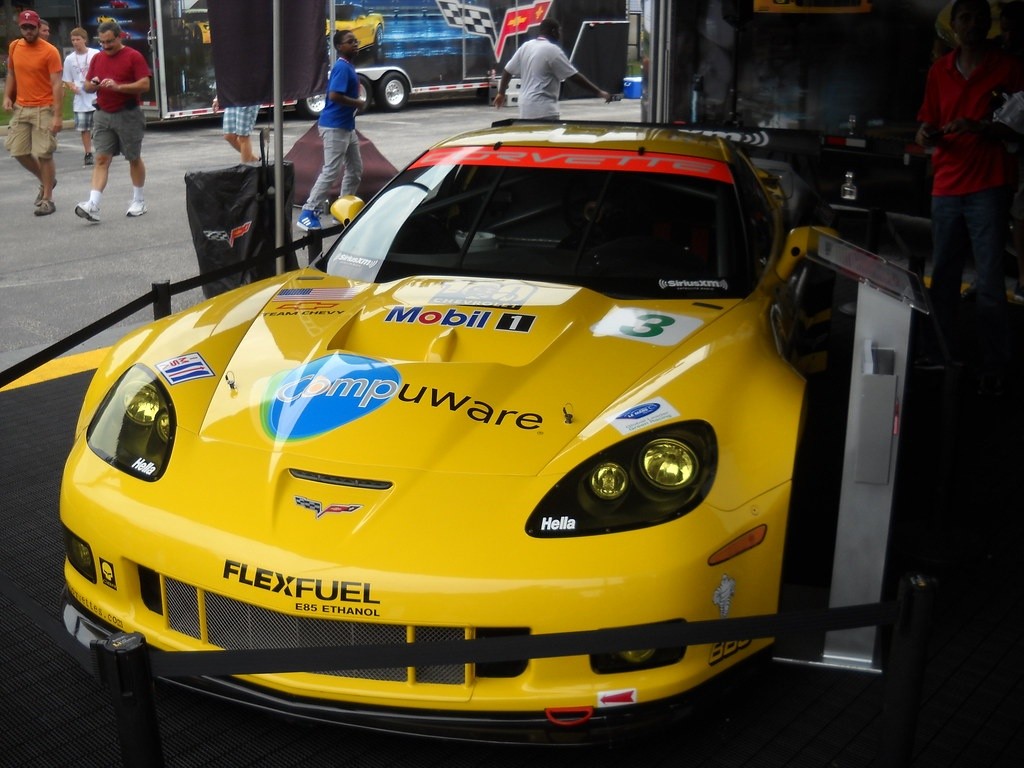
[[212, 95, 261, 165], [3, 10, 64, 216], [493, 18, 609, 125], [912, 0, 1019, 372], [297, 29, 366, 231], [60, 26, 102, 167], [74, 21, 154, 223], [37, 18, 50, 43]]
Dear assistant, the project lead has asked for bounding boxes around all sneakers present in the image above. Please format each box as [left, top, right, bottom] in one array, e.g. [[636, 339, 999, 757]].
[[297, 208, 322, 233], [74, 199, 100, 222], [32, 199, 58, 216], [83, 151, 94, 165], [125, 199, 147, 216], [33, 178, 59, 205]]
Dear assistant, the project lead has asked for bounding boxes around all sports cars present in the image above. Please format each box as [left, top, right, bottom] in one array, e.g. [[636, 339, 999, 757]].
[[54, 117, 848, 749]]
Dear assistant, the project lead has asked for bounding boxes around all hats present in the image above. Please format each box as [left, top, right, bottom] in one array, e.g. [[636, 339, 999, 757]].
[[18, 11, 40, 28]]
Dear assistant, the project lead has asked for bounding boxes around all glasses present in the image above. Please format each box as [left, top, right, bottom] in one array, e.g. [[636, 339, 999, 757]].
[[341, 37, 358, 45], [97, 35, 117, 44]]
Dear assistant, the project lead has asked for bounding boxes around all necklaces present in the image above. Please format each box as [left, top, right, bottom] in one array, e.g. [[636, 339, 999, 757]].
[[75, 47, 89, 80]]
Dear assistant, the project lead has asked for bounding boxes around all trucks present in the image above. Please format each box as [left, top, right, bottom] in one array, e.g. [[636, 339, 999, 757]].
[[627, 0, 1024, 362], [72, 0, 630, 134]]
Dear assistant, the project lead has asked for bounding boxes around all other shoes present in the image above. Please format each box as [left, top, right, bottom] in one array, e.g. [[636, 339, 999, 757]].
[[916, 343, 961, 372]]
